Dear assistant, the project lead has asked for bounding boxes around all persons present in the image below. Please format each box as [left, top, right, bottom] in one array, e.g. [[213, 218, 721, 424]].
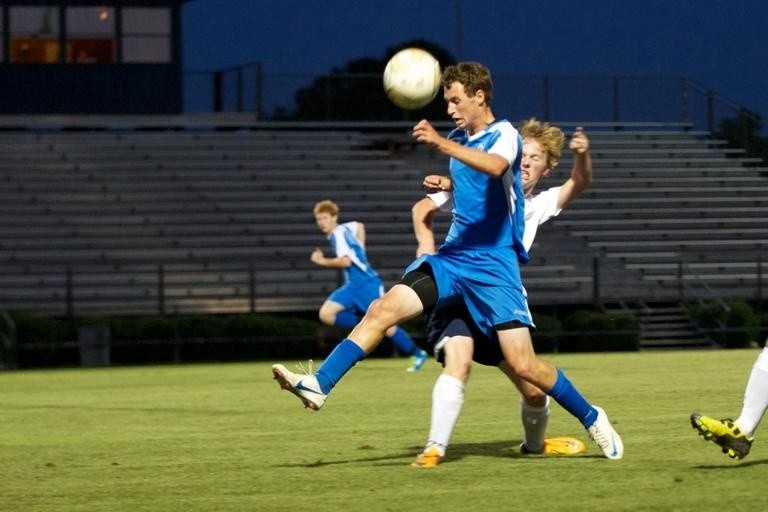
[[308, 199, 429, 374], [408, 116, 595, 470], [270, 60, 625, 459], [690, 338, 768, 460]]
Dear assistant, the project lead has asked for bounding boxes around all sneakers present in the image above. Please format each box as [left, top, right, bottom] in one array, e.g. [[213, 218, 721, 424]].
[[688, 410, 755, 461], [585, 403, 624, 461], [517, 436, 587, 456], [404, 345, 429, 374], [410, 441, 446, 468], [271, 358, 330, 411]]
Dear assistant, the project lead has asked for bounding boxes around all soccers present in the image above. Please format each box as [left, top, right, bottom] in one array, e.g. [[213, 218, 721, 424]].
[[383, 47, 442, 110]]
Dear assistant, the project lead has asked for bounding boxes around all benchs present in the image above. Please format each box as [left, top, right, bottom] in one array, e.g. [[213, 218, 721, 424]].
[[0, 113, 768, 350]]
